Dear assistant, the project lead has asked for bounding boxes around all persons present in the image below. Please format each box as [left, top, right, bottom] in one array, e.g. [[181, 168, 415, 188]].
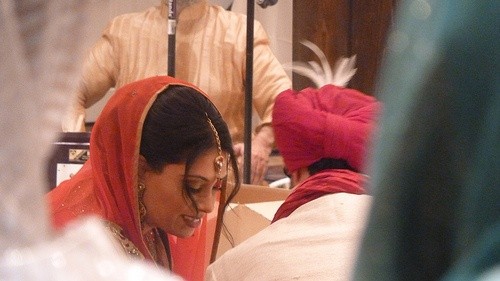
[[42, 76, 291, 281], [206, 85, 382, 281], [61, 0, 293, 185]]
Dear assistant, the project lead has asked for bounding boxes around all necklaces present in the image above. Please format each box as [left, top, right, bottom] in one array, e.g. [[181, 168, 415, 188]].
[[107, 221, 145, 259]]
